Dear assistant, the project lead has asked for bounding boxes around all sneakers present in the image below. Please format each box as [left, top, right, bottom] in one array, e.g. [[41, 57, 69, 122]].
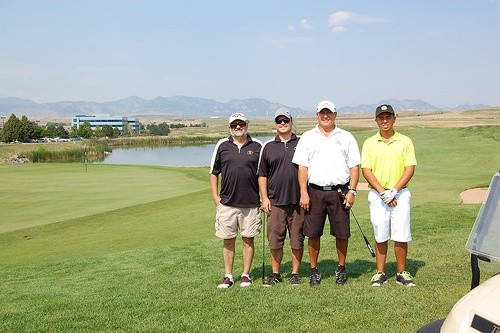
[[371, 271, 386, 286], [310, 273, 321, 287], [396, 270, 417, 286], [335, 265, 347, 285]]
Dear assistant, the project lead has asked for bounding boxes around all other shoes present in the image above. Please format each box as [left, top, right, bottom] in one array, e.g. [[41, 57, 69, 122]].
[[268, 273, 282, 285], [241, 276, 252, 287], [289, 269, 300, 287], [218, 277, 234, 289]]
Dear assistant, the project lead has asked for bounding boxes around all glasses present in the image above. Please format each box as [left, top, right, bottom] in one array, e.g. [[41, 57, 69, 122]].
[[276, 118, 290, 124], [230, 123, 246, 128]]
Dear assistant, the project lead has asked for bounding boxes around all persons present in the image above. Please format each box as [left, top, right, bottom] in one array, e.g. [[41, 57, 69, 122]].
[[210, 113, 263, 288], [256, 108, 305, 287], [291, 100, 361, 286], [361, 104, 417, 287]]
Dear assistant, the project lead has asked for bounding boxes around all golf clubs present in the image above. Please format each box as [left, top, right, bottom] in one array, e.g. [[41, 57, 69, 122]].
[[261, 210, 271, 287], [341, 193, 376, 258]]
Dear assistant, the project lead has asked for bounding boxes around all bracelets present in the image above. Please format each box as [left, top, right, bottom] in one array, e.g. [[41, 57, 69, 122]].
[[349, 189, 357, 195]]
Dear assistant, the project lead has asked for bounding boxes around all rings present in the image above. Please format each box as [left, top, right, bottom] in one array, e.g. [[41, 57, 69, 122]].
[[346, 203, 350, 206]]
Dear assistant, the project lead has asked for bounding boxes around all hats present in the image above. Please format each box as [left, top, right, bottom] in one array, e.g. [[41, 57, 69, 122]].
[[317, 100, 337, 113], [375, 104, 395, 117], [275, 108, 291, 119], [229, 113, 248, 126]]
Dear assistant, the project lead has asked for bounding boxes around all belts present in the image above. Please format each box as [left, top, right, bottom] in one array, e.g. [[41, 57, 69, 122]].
[[309, 182, 349, 191], [384, 186, 406, 190]]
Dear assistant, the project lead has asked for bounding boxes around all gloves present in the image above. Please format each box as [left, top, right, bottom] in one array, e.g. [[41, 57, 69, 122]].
[[381, 187, 398, 204]]
